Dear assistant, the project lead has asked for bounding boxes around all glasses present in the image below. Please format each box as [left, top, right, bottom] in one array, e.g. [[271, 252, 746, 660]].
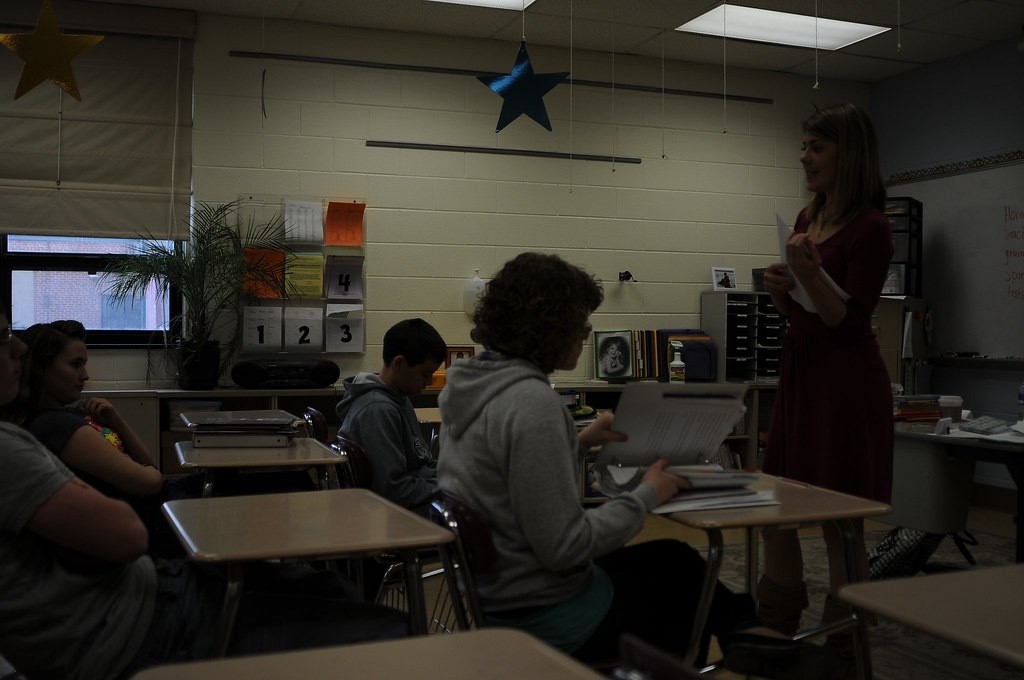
[[0, 325, 12, 341], [579, 323, 592, 341]]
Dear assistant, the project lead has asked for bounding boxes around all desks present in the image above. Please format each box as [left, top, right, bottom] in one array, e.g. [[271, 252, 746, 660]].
[[413, 408, 442, 455], [866, 422, 1024, 564], [601, 469, 894, 671], [128, 627, 607, 680], [836, 566, 1024, 680], [161, 486, 456, 661], [173, 440, 348, 497]]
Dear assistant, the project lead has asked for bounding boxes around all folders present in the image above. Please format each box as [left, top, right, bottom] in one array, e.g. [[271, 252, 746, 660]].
[[191, 434, 295, 448]]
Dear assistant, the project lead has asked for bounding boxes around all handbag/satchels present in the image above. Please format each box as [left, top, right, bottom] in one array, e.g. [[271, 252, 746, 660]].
[[867, 526, 979, 581]]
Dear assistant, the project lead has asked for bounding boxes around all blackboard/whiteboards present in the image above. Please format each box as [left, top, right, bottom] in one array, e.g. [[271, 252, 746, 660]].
[[885, 149, 1024, 373]]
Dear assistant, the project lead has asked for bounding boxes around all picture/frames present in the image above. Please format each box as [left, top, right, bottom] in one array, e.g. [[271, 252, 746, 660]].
[[445, 346, 474, 370], [712, 266, 738, 291]]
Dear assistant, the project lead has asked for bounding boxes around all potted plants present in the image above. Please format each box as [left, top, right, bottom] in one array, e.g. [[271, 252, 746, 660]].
[[105, 198, 305, 390]]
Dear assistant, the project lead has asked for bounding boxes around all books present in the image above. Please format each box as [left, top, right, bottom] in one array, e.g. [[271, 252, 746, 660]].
[[893, 395, 1008, 435], [583, 383, 781, 516]]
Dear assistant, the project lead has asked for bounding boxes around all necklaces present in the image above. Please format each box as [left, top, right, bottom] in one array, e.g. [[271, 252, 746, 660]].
[[817, 203, 848, 238]]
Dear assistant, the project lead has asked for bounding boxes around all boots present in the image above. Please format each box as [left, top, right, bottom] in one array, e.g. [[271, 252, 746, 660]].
[[757, 574, 809, 635], [821, 593, 873, 680]]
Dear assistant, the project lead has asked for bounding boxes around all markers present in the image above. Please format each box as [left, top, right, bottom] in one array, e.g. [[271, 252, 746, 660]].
[[972, 355, 989, 358]]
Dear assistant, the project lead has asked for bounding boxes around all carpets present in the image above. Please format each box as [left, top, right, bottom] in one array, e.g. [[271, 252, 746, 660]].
[[694, 528, 1024, 680]]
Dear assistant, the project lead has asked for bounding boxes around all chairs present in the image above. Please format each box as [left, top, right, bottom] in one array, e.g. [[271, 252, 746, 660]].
[[426, 485, 619, 674], [330, 434, 475, 633], [303, 403, 331, 490]]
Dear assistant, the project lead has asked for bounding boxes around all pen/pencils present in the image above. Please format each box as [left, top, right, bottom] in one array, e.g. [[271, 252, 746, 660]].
[[779, 478, 811, 488]]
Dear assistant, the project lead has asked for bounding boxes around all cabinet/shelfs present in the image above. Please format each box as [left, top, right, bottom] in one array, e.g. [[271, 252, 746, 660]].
[[83, 380, 779, 503], [878, 296, 926, 384], [700, 291, 785, 383], [880, 196, 923, 299]]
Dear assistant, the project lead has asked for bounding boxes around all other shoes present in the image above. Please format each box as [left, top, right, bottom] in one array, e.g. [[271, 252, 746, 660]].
[[724, 633, 850, 680]]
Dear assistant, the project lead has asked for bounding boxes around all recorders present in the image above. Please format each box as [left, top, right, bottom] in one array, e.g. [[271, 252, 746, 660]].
[[231, 356, 340, 390]]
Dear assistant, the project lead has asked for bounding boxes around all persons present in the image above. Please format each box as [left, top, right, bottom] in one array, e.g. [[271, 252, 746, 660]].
[[754, 103, 893, 660], [336, 319, 447, 521], [0, 310, 406, 680], [437, 254, 802, 680], [723, 273, 731, 288], [599, 341, 624, 373]]
